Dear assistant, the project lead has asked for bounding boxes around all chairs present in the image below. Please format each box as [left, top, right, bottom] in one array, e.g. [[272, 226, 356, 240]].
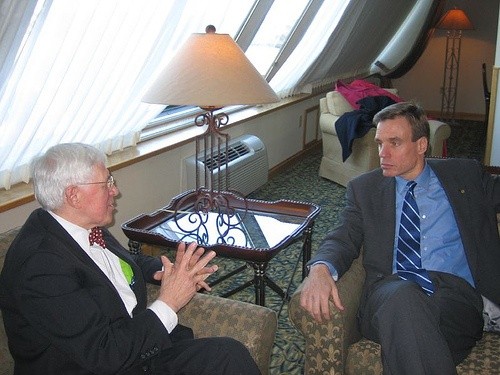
[[481, 63, 491, 146]]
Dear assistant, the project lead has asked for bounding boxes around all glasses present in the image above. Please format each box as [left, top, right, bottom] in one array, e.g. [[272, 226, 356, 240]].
[[68, 175, 114, 190]]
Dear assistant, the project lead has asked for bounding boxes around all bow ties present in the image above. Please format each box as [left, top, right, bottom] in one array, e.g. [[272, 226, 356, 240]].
[[88, 227, 106, 249]]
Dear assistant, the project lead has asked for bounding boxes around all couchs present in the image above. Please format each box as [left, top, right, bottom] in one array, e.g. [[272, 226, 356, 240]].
[[317, 87, 452, 190], [0, 226, 277, 375], [288, 158, 500, 375]]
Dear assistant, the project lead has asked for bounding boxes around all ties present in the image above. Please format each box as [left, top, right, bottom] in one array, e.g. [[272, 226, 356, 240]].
[[396, 182, 435, 297]]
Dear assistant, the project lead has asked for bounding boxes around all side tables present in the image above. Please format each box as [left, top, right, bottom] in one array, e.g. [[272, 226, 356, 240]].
[[121, 187, 322, 333]]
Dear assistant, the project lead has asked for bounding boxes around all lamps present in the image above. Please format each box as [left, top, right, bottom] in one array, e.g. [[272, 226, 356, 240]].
[[141, 25, 283, 243], [431, 7, 475, 128]]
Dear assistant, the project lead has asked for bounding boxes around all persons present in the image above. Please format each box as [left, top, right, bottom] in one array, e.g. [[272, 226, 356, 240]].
[[300, 102, 500, 375], [0, 142, 262, 375]]
